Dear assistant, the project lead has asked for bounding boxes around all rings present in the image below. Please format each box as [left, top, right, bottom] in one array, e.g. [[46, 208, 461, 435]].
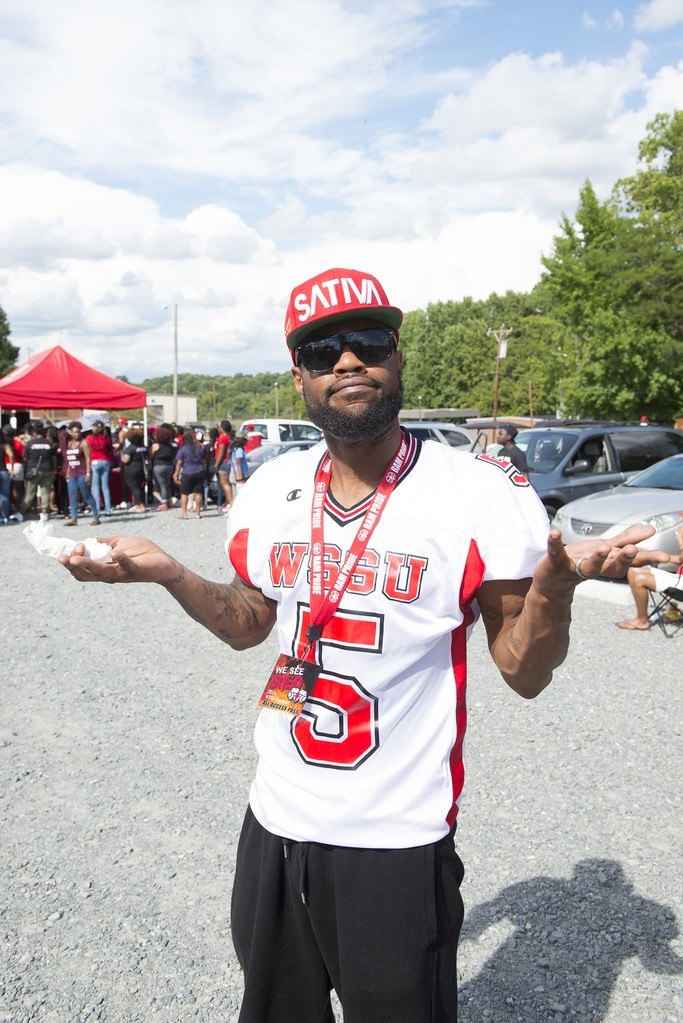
[[575, 556, 589, 580]]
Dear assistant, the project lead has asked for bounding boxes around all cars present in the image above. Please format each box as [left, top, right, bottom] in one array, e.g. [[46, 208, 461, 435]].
[[477, 416, 683, 528], [550, 453, 683, 574], [479, 425, 561, 465], [395, 420, 481, 456], [206, 440, 318, 505]]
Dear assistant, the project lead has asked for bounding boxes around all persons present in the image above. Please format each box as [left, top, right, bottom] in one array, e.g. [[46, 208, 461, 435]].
[[614, 526, 683, 631], [0, 410, 266, 527], [58, 266, 674, 1023], [496, 423, 530, 482]]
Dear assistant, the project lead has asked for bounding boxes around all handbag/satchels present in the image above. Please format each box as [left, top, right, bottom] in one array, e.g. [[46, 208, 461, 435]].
[[25, 467, 38, 480], [144, 464, 150, 480]]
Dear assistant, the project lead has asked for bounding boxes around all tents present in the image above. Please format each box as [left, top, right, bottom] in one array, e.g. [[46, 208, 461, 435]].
[[0, 345, 148, 508]]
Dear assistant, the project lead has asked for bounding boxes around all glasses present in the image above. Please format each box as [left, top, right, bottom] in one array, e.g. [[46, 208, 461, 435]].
[[296, 329, 397, 373]]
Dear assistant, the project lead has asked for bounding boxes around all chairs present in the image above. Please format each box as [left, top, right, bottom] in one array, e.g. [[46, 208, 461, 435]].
[[581, 442, 606, 472]]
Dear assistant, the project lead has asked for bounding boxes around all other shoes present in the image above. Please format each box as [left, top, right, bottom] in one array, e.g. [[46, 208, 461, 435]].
[[0, 496, 232, 527]]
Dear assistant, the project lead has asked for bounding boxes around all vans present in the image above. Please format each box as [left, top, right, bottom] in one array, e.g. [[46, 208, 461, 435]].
[[240, 418, 326, 447]]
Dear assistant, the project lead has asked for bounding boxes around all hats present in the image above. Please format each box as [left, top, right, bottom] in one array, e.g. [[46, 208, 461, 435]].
[[641, 416, 647, 423], [91, 420, 104, 427], [500, 423, 518, 444], [284, 268, 403, 368], [115, 417, 128, 425], [246, 424, 254, 430]]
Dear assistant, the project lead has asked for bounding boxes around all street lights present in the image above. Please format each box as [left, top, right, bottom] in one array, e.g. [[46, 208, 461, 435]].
[[163, 303, 177, 426]]
